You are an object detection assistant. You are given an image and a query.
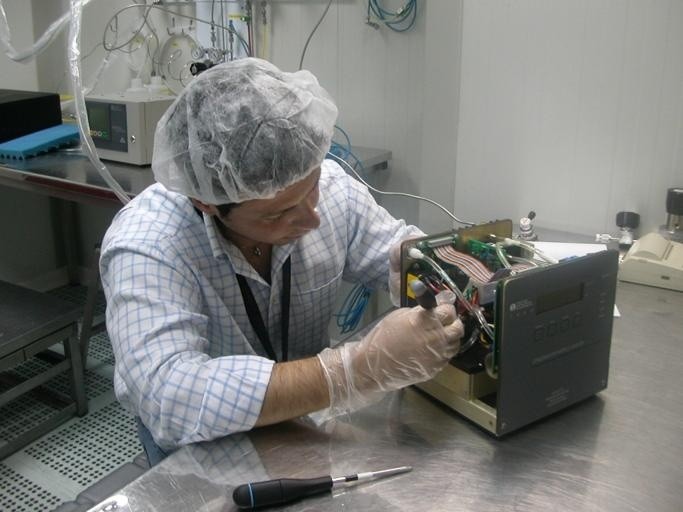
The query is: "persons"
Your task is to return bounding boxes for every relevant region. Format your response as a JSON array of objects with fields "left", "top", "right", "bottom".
[
  {"left": 148, "top": 430, "right": 314, "bottom": 485},
  {"left": 96, "top": 56, "right": 470, "bottom": 456}
]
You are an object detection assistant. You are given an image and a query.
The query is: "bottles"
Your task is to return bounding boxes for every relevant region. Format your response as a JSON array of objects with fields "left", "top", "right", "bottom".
[
  {"left": 516, "top": 217, "right": 538, "bottom": 242},
  {"left": 149, "top": 76, "right": 167, "bottom": 96},
  {"left": 127, "top": 79, "right": 148, "bottom": 99}
]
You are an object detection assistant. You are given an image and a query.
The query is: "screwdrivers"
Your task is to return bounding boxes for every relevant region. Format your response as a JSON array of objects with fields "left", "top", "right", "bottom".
[{"left": 232, "top": 463, "right": 414, "bottom": 509}]
